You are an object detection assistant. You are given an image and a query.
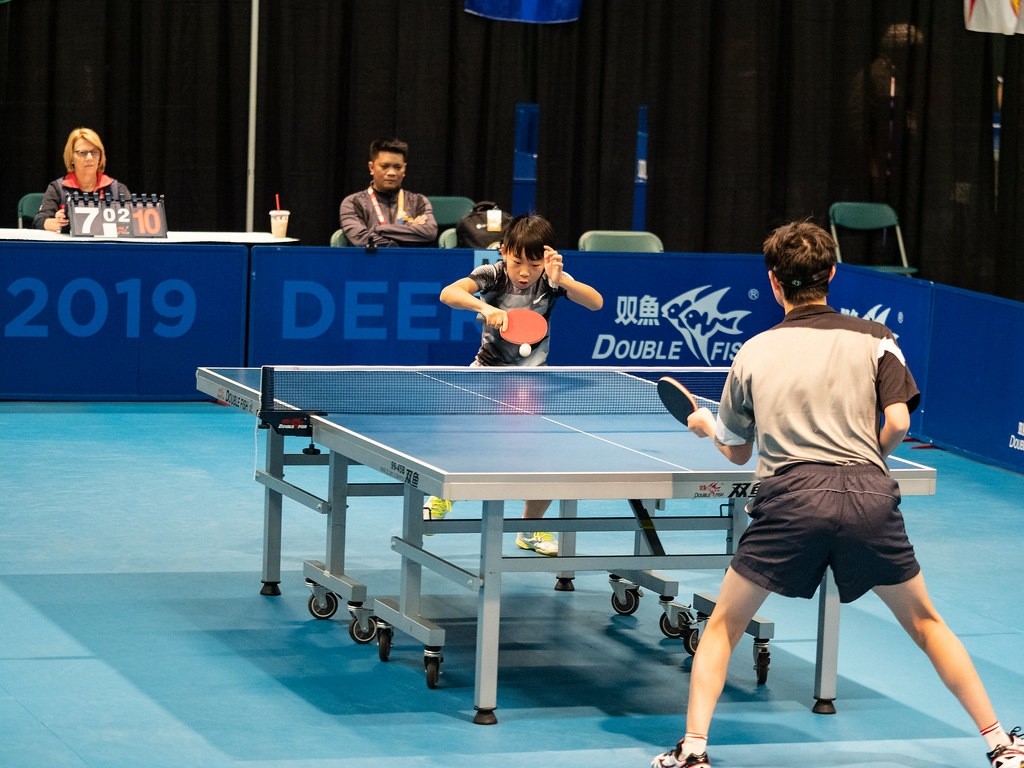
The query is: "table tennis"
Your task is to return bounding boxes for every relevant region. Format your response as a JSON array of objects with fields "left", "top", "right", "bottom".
[{"left": 519, "top": 343, "right": 532, "bottom": 358}]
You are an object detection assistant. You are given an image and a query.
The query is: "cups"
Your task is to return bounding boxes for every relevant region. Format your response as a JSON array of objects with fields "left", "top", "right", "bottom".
[
  {"left": 487, "top": 210, "right": 502, "bottom": 230},
  {"left": 269, "top": 210, "right": 290, "bottom": 238}
]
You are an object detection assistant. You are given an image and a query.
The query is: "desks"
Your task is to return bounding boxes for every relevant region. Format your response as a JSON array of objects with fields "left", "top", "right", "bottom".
[{"left": 197, "top": 365, "right": 938, "bottom": 726}]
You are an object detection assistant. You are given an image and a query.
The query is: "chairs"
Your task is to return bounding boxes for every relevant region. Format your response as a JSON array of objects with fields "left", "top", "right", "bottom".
[
  {"left": 829, "top": 201, "right": 918, "bottom": 277},
  {"left": 19, "top": 193, "right": 46, "bottom": 229},
  {"left": 329, "top": 197, "right": 475, "bottom": 248},
  {"left": 579, "top": 231, "right": 664, "bottom": 253}
]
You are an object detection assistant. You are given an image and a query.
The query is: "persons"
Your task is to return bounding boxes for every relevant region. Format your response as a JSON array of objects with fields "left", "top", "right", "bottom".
[
  {"left": 423, "top": 214, "right": 603, "bottom": 556},
  {"left": 340, "top": 138, "right": 438, "bottom": 247},
  {"left": 650, "top": 221, "right": 1024, "bottom": 768},
  {"left": 33, "top": 127, "right": 131, "bottom": 233}
]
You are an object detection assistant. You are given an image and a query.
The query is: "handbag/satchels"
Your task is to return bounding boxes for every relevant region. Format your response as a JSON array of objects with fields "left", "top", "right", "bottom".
[{"left": 456, "top": 201, "right": 514, "bottom": 250}]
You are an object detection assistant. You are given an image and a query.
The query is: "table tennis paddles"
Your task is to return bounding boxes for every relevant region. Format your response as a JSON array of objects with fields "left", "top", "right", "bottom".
[
  {"left": 475, "top": 308, "right": 549, "bottom": 345},
  {"left": 656, "top": 375, "right": 699, "bottom": 428}
]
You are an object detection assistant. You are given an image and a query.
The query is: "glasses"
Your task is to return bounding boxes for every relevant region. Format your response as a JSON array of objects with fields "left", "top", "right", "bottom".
[{"left": 73, "top": 150, "right": 100, "bottom": 158}]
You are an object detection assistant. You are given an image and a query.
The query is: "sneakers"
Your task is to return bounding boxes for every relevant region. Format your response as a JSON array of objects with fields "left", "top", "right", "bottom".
[
  {"left": 422, "top": 495, "right": 457, "bottom": 536},
  {"left": 516, "top": 531, "right": 558, "bottom": 556},
  {"left": 987, "top": 726, "right": 1024, "bottom": 768},
  {"left": 651, "top": 739, "right": 711, "bottom": 768}
]
